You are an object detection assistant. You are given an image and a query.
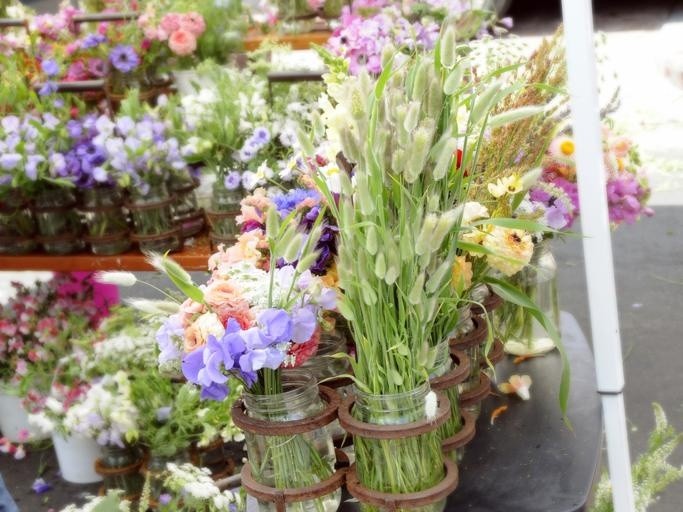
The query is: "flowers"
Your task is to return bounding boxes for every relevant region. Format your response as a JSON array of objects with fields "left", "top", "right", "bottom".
[{"left": 1, "top": 0, "right": 681, "bottom": 511}]
